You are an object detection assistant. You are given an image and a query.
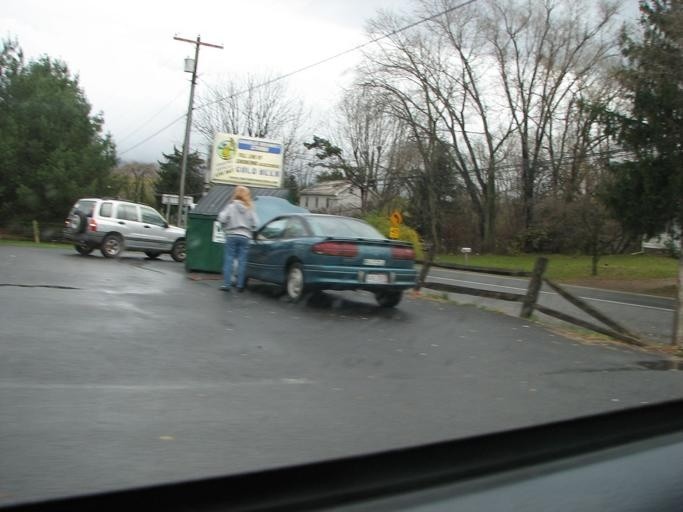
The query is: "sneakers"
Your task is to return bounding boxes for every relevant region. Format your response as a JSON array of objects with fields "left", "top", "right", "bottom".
[{"left": 219, "top": 285, "right": 244, "bottom": 293}]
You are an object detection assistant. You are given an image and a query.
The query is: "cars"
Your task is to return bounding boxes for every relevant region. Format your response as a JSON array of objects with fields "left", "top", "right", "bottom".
[{"left": 224, "top": 193, "right": 420, "bottom": 311}]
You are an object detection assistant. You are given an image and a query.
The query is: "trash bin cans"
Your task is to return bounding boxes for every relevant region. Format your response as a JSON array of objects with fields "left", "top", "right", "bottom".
[{"left": 187, "top": 185, "right": 295, "bottom": 275}]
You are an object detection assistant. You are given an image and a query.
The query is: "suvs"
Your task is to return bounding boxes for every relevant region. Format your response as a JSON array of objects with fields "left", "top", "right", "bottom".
[{"left": 63, "top": 197, "right": 191, "bottom": 262}]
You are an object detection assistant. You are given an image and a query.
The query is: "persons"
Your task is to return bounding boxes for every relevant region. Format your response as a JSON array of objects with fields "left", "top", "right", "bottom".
[{"left": 216, "top": 186, "right": 259, "bottom": 293}]
